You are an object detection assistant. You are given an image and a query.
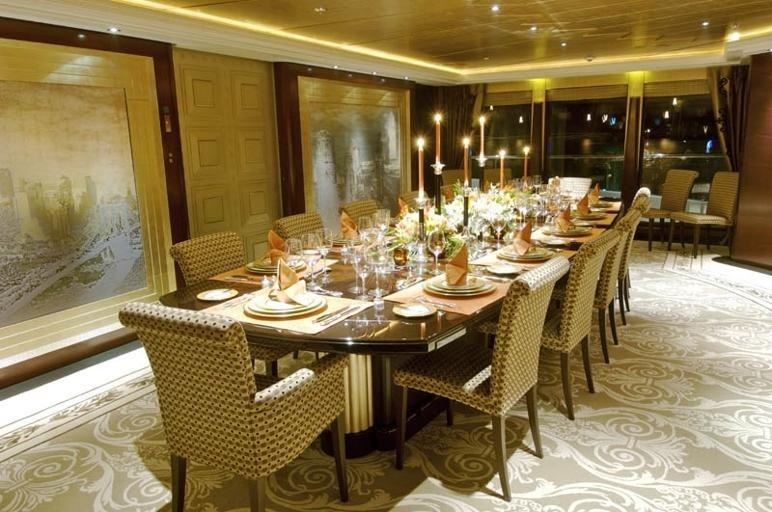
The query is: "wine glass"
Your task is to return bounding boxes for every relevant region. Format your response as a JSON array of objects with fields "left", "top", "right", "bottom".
[{"left": 300, "top": 176, "right": 565, "bottom": 304}]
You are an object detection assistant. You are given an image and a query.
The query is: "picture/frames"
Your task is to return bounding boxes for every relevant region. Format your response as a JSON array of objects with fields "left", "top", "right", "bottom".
[
  {"left": 271, "top": 59, "right": 418, "bottom": 218},
  {"left": 1, "top": 15, "right": 193, "bottom": 389}
]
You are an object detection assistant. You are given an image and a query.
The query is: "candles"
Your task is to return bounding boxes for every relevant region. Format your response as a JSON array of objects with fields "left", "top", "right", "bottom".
[{"left": 411, "top": 110, "right": 531, "bottom": 199}]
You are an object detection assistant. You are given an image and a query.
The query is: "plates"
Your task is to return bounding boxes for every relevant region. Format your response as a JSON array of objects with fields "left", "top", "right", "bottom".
[
  {"left": 423, "top": 198, "right": 611, "bottom": 299},
  {"left": 391, "top": 300, "right": 437, "bottom": 322},
  {"left": 197, "top": 232, "right": 359, "bottom": 320}
]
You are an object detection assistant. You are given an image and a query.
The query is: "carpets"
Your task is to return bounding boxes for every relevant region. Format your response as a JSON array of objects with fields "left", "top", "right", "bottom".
[{"left": 2, "top": 238, "right": 770, "bottom": 512}]
